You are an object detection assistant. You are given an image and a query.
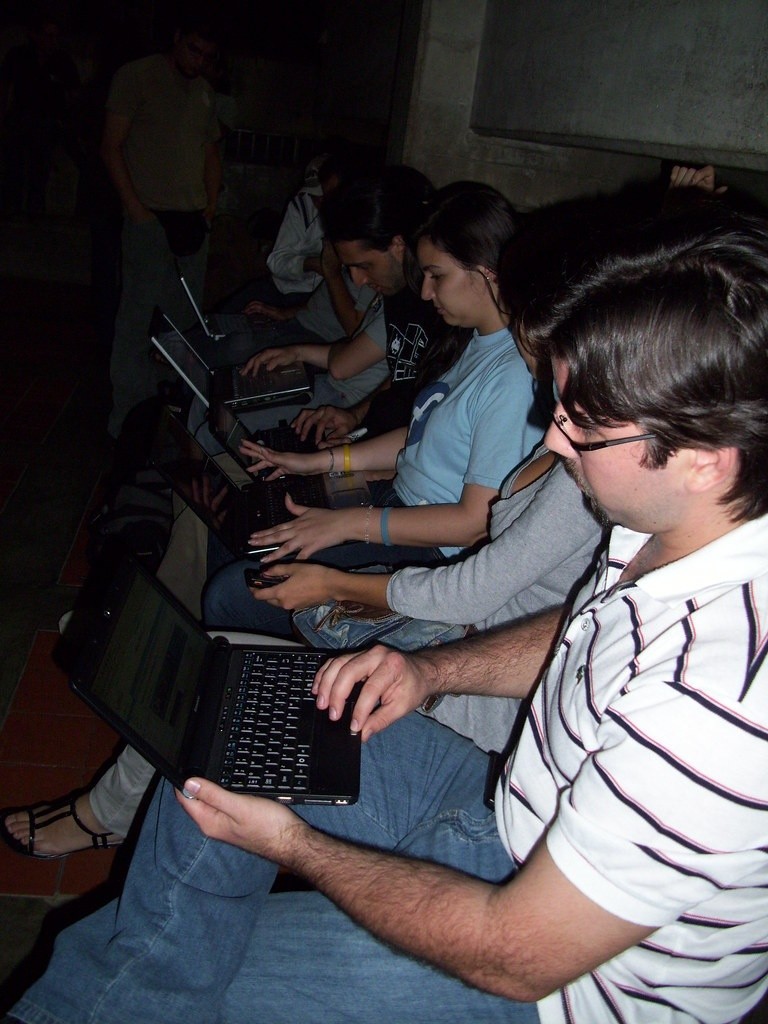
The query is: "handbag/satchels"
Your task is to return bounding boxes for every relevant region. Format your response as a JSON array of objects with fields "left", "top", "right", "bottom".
[{"left": 151, "top": 209, "right": 206, "bottom": 257}]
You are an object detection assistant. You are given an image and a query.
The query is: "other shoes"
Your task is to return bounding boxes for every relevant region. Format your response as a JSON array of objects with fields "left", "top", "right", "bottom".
[{"left": 59, "top": 609, "right": 111, "bottom": 644}]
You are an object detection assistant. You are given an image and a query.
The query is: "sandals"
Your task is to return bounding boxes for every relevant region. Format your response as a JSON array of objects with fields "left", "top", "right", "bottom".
[{"left": 0, "top": 794, "right": 144, "bottom": 861}]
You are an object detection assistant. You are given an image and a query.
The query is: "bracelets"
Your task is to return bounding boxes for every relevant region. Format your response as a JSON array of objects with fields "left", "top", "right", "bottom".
[
  {"left": 326, "top": 447, "right": 334, "bottom": 473},
  {"left": 364, "top": 505, "right": 374, "bottom": 544},
  {"left": 343, "top": 444, "right": 351, "bottom": 473},
  {"left": 381, "top": 507, "right": 393, "bottom": 547}
]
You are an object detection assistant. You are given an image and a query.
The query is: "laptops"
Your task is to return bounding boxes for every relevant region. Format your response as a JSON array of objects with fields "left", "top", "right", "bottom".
[{"left": 70, "top": 249, "right": 376, "bottom": 806}]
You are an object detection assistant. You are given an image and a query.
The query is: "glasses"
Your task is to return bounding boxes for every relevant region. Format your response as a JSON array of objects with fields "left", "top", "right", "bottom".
[{"left": 532, "top": 377, "right": 661, "bottom": 459}]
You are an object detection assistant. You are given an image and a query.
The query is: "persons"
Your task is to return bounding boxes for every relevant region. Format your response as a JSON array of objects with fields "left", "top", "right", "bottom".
[
  {"left": 0, "top": 124, "right": 730, "bottom": 866},
  {"left": 103, "top": 17, "right": 221, "bottom": 440},
  {"left": 0, "top": 194, "right": 768, "bottom": 1024}
]
